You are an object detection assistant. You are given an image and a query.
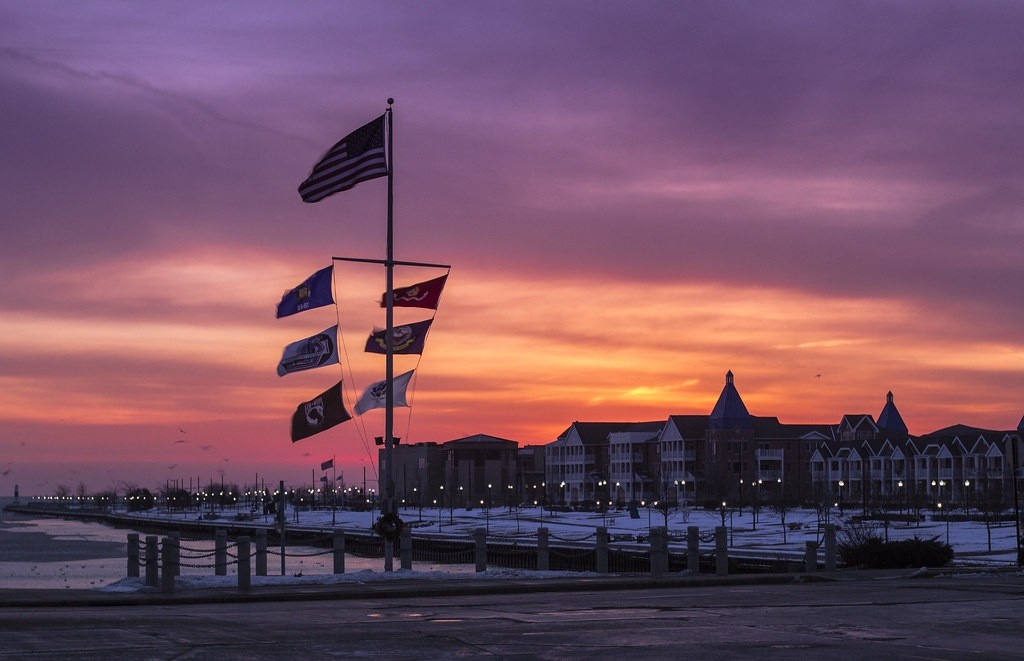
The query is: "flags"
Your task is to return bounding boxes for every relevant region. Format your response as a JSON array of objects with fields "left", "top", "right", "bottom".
[
  {"left": 364, "top": 318, "right": 433, "bottom": 357},
  {"left": 336, "top": 476, "right": 343, "bottom": 480},
  {"left": 320, "top": 476, "right": 328, "bottom": 482},
  {"left": 393, "top": 437, "right": 401, "bottom": 445},
  {"left": 321, "top": 459, "right": 334, "bottom": 471},
  {"left": 374, "top": 436, "right": 384, "bottom": 445},
  {"left": 378, "top": 274, "right": 449, "bottom": 310},
  {"left": 353, "top": 368, "right": 415, "bottom": 417},
  {"left": 275, "top": 264, "right": 335, "bottom": 319},
  {"left": 297, "top": 110, "right": 389, "bottom": 203},
  {"left": 275, "top": 324, "right": 342, "bottom": 378},
  {"left": 288, "top": 380, "right": 352, "bottom": 443}
]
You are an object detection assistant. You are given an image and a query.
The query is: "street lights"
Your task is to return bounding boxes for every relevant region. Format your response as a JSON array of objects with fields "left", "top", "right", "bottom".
[
  {"left": 839, "top": 480, "right": 844, "bottom": 517},
  {"left": 508, "top": 485, "right": 513, "bottom": 514},
  {"left": 640, "top": 499, "right": 657, "bottom": 535},
  {"left": 137, "top": 496, "right": 145, "bottom": 514},
  {"left": 598, "top": 480, "right": 607, "bottom": 526},
  {"left": 932, "top": 480, "right": 936, "bottom": 513},
  {"left": 965, "top": 480, "right": 970, "bottom": 516},
  {"left": 681, "top": 480, "right": 686, "bottom": 511},
  {"left": 739, "top": 479, "right": 743, "bottom": 516},
  {"left": 557, "top": 480, "right": 565, "bottom": 507},
  {"left": 752, "top": 482, "right": 756, "bottom": 530},
  {"left": 776, "top": 477, "right": 781, "bottom": 513},
  {"left": 758, "top": 479, "right": 762, "bottom": 509},
  {"left": 722, "top": 502, "right": 726, "bottom": 526},
  {"left": 440, "top": 485, "right": 444, "bottom": 511},
  {"left": 616, "top": 482, "right": 620, "bottom": 501},
  {"left": 674, "top": 480, "right": 678, "bottom": 511},
  {"left": 898, "top": 481, "right": 903, "bottom": 521},
  {"left": 459, "top": 486, "right": 463, "bottom": 508},
  {"left": 488, "top": 483, "right": 492, "bottom": 511},
  {"left": 939, "top": 480, "right": 945, "bottom": 516},
  {"left": 166, "top": 496, "right": 176, "bottom": 515}
]
